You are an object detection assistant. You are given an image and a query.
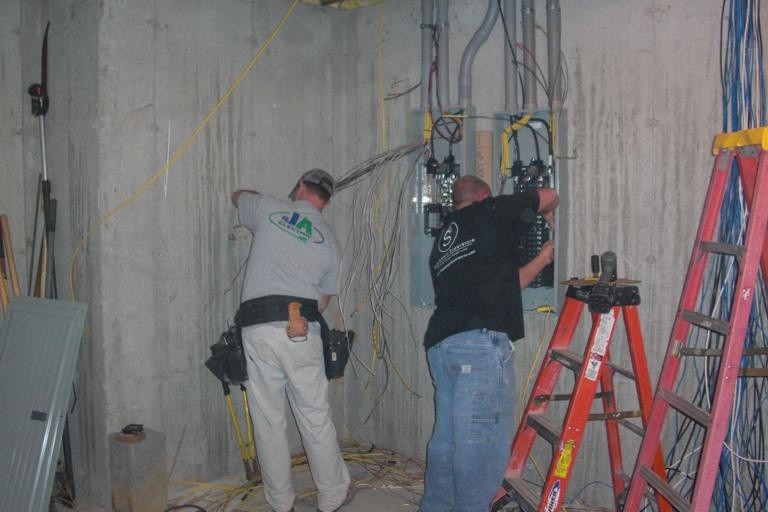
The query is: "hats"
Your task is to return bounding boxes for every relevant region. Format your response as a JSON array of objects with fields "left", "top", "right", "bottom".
[{"left": 288, "top": 169, "right": 333, "bottom": 198}]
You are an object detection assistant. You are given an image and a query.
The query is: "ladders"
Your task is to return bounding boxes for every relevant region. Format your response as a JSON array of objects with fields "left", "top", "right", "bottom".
[
  {"left": 623, "top": 127, "right": 768, "bottom": 512},
  {"left": 488, "top": 274, "right": 671, "bottom": 510}
]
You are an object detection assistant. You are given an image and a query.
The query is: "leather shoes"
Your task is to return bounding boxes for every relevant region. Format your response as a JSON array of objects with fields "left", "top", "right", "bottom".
[{"left": 317, "top": 488, "right": 353, "bottom": 512}]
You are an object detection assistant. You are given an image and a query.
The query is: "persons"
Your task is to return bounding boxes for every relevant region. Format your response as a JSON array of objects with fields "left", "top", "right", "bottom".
[
  {"left": 229, "top": 168, "right": 358, "bottom": 512},
  {"left": 417, "top": 174, "right": 561, "bottom": 511}
]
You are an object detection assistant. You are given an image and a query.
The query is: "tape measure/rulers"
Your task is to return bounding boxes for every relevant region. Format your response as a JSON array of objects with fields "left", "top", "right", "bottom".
[{"left": 123, "top": 424, "right": 143, "bottom": 433}]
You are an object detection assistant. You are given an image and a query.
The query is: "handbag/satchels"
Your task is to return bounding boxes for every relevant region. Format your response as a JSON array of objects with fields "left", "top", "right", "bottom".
[
  {"left": 323, "top": 328, "right": 354, "bottom": 382},
  {"left": 205, "top": 324, "right": 248, "bottom": 385}
]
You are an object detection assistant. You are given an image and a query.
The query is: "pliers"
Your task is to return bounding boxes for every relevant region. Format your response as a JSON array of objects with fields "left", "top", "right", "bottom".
[{"left": 222, "top": 383, "right": 261, "bottom": 485}]
